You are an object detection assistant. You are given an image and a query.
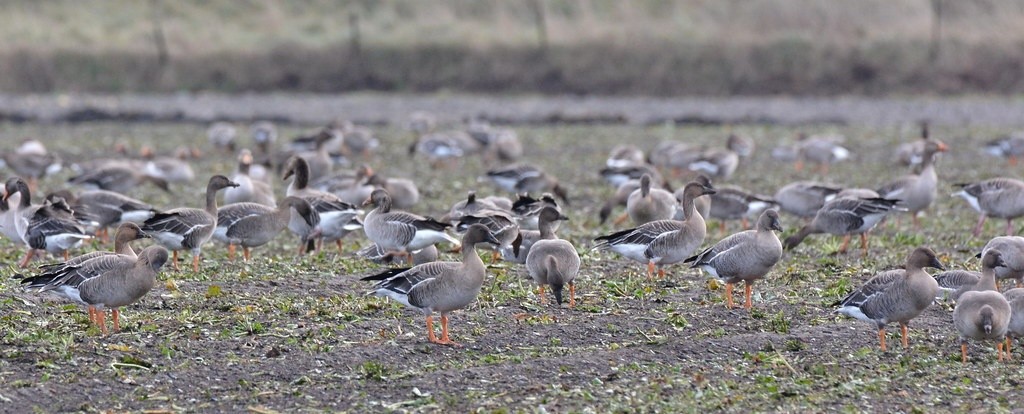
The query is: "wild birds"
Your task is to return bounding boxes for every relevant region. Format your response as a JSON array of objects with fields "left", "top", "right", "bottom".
[{"left": 0, "top": 112, "right": 1024, "bottom": 368}]
